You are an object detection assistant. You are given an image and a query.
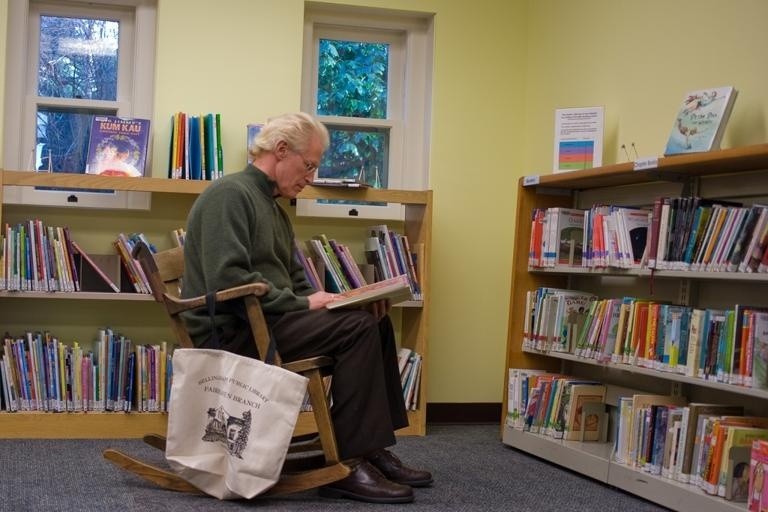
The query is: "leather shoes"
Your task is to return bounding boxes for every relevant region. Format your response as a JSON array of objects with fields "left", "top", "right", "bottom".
[
  {"left": 369, "top": 449, "right": 435, "bottom": 486},
  {"left": 319, "top": 458, "right": 415, "bottom": 503}
]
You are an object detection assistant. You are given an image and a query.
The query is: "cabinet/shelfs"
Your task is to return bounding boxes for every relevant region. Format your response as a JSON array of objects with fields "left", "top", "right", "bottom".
[
  {"left": 0, "top": 167, "right": 435, "bottom": 440},
  {"left": 499, "top": 141, "right": 768, "bottom": 512}
]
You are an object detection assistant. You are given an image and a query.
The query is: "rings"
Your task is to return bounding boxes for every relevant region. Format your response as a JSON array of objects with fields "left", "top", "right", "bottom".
[{"left": 331, "top": 294, "right": 334, "bottom": 298}]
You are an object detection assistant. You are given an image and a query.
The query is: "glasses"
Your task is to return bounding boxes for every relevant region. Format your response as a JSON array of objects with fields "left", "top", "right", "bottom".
[{"left": 300, "top": 153, "right": 319, "bottom": 178}]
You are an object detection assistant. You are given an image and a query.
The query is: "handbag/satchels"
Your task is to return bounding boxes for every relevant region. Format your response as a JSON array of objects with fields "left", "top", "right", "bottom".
[{"left": 165, "top": 348, "right": 309, "bottom": 501}]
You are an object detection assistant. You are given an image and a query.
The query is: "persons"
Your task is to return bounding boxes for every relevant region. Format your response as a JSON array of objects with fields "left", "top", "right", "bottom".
[{"left": 174, "top": 110, "right": 437, "bottom": 505}]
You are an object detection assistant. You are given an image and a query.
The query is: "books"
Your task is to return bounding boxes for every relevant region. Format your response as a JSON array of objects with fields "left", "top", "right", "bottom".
[
  {"left": 295, "top": 234, "right": 368, "bottom": 294},
  {"left": 573, "top": 297, "right": 766, "bottom": 390},
  {"left": 2, "top": 219, "right": 80, "bottom": 292},
  {"left": 523, "top": 288, "right": 599, "bottom": 352},
  {"left": 647, "top": 196, "right": 765, "bottom": 273},
  {"left": 308, "top": 176, "right": 372, "bottom": 190},
  {"left": 92, "top": 328, "right": 171, "bottom": 414},
  {"left": 114, "top": 232, "right": 157, "bottom": 295},
  {"left": 71, "top": 241, "right": 120, "bottom": 293},
  {"left": 299, "top": 375, "right": 332, "bottom": 413},
  {"left": 504, "top": 368, "right": 607, "bottom": 440},
  {"left": 582, "top": 203, "right": 652, "bottom": 268},
  {"left": 363, "top": 225, "right": 421, "bottom": 301},
  {"left": 615, "top": 394, "right": 767, "bottom": 511},
  {"left": 396, "top": 347, "right": 422, "bottom": 412},
  {"left": 528, "top": 208, "right": 585, "bottom": 268},
  {"left": 168, "top": 228, "right": 187, "bottom": 281},
  {"left": 242, "top": 120, "right": 270, "bottom": 166},
  {"left": 164, "top": 109, "right": 226, "bottom": 181},
  {"left": 80, "top": 113, "right": 151, "bottom": 180},
  {"left": 326, "top": 273, "right": 415, "bottom": 309},
  {"left": 1, "top": 331, "right": 99, "bottom": 413},
  {"left": 663, "top": 85, "right": 740, "bottom": 158},
  {"left": 358, "top": 264, "right": 375, "bottom": 284},
  {"left": 549, "top": 104, "right": 606, "bottom": 175}
]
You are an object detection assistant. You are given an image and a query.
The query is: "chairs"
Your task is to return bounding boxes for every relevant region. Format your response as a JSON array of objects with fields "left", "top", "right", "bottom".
[{"left": 102, "top": 243, "right": 351, "bottom": 500}]
